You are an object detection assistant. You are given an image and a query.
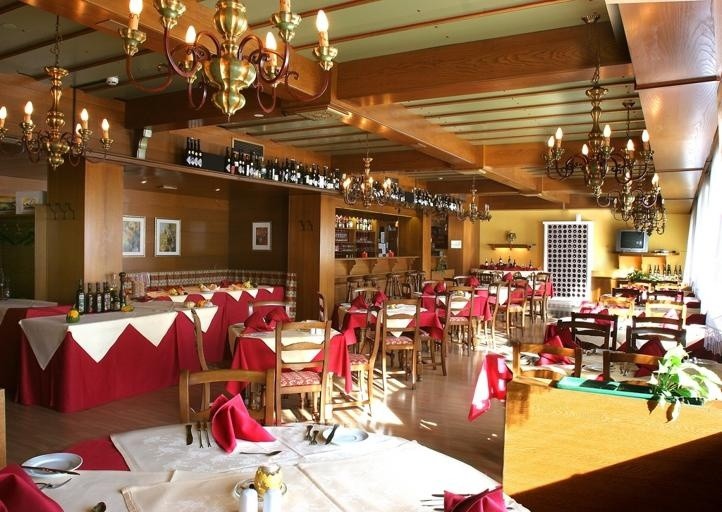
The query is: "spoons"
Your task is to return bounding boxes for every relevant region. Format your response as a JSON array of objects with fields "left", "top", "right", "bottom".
[{"left": 91, "top": 502, "right": 106, "bottom": 512}]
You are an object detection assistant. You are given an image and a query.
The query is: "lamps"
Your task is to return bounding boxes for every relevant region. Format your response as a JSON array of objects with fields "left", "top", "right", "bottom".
[
  {"left": 0, "top": 14, "right": 114, "bottom": 172},
  {"left": 456, "top": 187, "right": 492, "bottom": 223},
  {"left": 619, "top": 157, "right": 666, "bottom": 236},
  {"left": 342, "top": 133, "right": 395, "bottom": 209},
  {"left": 539, "top": 13, "right": 651, "bottom": 198},
  {"left": 596, "top": 102, "right": 660, "bottom": 217},
  {"left": 117, "top": 0, "right": 338, "bottom": 123}
]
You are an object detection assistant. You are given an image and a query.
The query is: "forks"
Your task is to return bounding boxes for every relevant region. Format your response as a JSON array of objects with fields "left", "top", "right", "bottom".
[
  {"left": 239, "top": 450, "right": 282, "bottom": 456},
  {"left": 309, "top": 430, "right": 319, "bottom": 446},
  {"left": 304, "top": 425, "right": 314, "bottom": 441}
]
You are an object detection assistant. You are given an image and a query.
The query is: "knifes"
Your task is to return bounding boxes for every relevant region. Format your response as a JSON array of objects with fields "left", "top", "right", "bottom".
[
  {"left": 21, "top": 466, "right": 80, "bottom": 475},
  {"left": 186, "top": 425, "right": 193, "bottom": 445},
  {"left": 326, "top": 424, "right": 339, "bottom": 444}
]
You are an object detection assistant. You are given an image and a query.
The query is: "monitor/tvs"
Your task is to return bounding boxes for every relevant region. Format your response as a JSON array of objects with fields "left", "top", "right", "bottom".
[{"left": 616, "top": 229, "right": 648, "bottom": 253}]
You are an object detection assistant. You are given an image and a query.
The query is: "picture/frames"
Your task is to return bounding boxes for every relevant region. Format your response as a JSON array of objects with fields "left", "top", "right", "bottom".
[
  {"left": 153, "top": 216, "right": 182, "bottom": 256},
  {"left": 251, "top": 221, "right": 273, "bottom": 252},
  {"left": 121, "top": 215, "right": 147, "bottom": 258}
]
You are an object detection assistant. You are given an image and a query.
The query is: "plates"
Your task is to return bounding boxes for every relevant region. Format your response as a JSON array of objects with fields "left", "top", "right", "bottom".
[
  {"left": 232, "top": 480, "right": 288, "bottom": 507},
  {"left": 22, "top": 453, "right": 84, "bottom": 478},
  {"left": 322, "top": 428, "right": 369, "bottom": 445}
]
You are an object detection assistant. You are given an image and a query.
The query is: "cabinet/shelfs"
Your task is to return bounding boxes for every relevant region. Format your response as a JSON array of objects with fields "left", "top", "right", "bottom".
[{"left": 334, "top": 218, "right": 378, "bottom": 258}]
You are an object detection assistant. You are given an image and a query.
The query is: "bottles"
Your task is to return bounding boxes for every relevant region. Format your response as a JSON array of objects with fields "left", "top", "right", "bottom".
[
  {"left": 224, "top": 146, "right": 464, "bottom": 260},
  {"left": 185, "top": 136, "right": 203, "bottom": 168},
  {"left": 479, "top": 255, "right": 533, "bottom": 286},
  {"left": 75, "top": 272, "right": 121, "bottom": 315},
  {"left": 649, "top": 265, "right": 682, "bottom": 281},
  {"left": 0, "top": 263, "right": 12, "bottom": 298}
]
